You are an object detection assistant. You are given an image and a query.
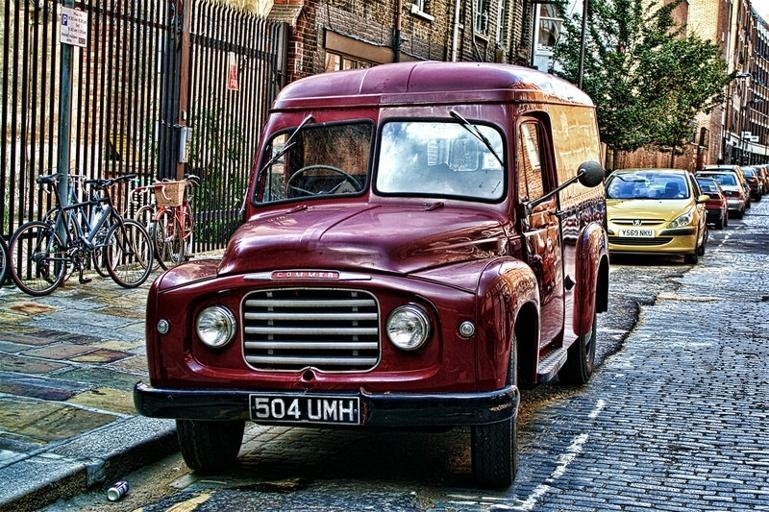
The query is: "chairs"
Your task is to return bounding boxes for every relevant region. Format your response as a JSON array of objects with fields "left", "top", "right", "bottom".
[{"left": 664, "top": 178, "right": 682, "bottom": 200}]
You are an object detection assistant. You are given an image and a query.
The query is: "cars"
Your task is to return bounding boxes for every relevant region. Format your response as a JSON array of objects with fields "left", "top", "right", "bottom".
[
  {"left": 696, "top": 177, "right": 733, "bottom": 230},
  {"left": 604, "top": 167, "right": 710, "bottom": 265},
  {"left": 694, "top": 171, "right": 749, "bottom": 219},
  {"left": 740, "top": 163, "right": 769, "bottom": 202},
  {"left": 695, "top": 163, "right": 756, "bottom": 209}
]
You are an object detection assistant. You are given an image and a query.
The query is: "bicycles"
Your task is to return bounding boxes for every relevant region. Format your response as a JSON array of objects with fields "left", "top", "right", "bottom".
[
  {"left": 151, "top": 173, "right": 200, "bottom": 272},
  {"left": 9, "top": 174, "right": 153, "bottom": 296},
  {"left": 0, "top": 235, "right": 9, "bottom": 290},
  {"left": 128, "top": 185, "right": 189, "bottom": 274},
  {"left": 36, "top": 174, "right": 124, "bottom": 287}
]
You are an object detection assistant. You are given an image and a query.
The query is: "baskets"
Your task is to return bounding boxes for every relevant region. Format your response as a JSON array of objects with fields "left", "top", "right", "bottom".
[{"left": 152, "top": 180, "right": 186, "bottom": 208}]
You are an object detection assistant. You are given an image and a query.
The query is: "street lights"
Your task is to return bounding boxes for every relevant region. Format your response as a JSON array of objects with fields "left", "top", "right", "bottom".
[
  {"left": 721, "top": 74, "right": 751, "bottom": 166},
  {"left": 740, "top": 99, "right": 764, "bottom": 165}
]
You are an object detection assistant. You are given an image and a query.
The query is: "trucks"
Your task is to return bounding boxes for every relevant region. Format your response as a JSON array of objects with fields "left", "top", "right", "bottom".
[{"left": 132, "top": 59, "right": 612, "bottom": 491}]
